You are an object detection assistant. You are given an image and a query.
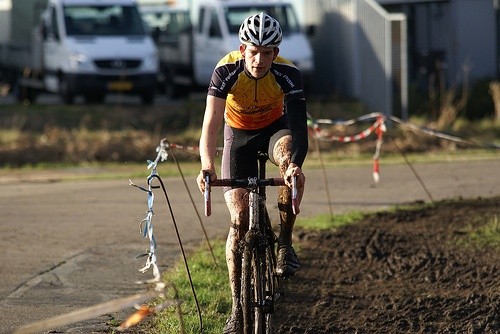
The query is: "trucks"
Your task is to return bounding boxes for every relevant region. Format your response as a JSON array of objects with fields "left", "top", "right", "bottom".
[{"left": 0, "top": 0, "right": 164, "bottom": 107}]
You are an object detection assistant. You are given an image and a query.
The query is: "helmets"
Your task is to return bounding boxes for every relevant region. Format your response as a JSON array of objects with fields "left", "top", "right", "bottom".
[{"left": 238, "top": 12, "right": 283, "bottom": 48}]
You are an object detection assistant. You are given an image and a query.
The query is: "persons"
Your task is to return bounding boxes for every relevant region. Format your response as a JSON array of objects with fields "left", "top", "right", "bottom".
[{"left": 196, "top": 12, "right": 308, "bottom": 334}]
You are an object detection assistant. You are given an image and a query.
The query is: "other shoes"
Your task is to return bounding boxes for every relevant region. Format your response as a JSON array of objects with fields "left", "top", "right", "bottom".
[
  {"left": 223, "top": 311, "right": 243, "bottom": 334},
  {"left": 275, "top": 246, "right": 300, "bottom": 276}
]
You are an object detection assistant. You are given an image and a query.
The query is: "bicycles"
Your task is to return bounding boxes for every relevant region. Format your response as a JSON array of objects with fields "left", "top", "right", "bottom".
[{"left": 204, "top": 174, "right": 300, "bottom": 334}]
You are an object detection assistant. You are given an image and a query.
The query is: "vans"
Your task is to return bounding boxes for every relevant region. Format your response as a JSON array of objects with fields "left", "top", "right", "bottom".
[{"left": 140, "top": 1, "right": 317, "bottom": 97}]
